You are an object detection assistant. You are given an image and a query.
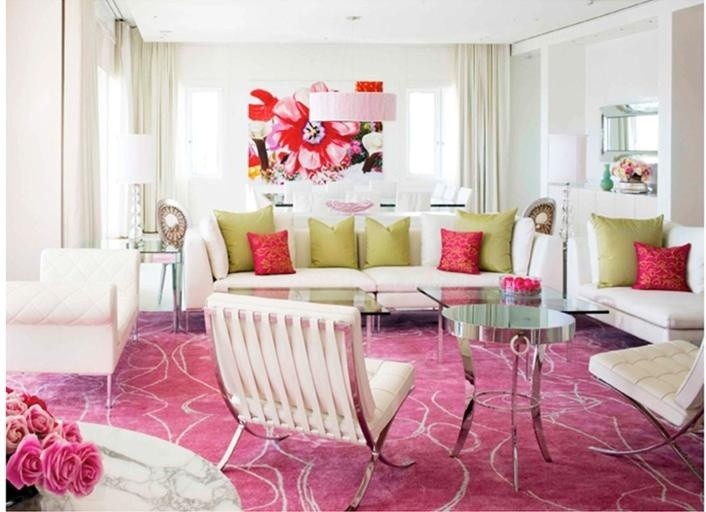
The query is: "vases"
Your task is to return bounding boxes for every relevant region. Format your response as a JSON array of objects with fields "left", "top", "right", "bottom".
[{"left": 618, "top": 181, "right": 649, "bottom": 195}]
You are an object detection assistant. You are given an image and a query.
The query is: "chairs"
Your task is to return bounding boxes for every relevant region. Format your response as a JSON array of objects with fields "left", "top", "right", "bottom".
[
  {"left": 431, "top": 182, "right": 475, "bottom": 202},
  {"left": 206, "top": 295, "right": 419, "bottom": 509},
  {"left": 590, "top": 319, "right": 702, "bottom": 486}
]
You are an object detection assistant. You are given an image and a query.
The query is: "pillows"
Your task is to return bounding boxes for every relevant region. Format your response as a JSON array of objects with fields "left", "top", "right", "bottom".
[
  {"left": 363, "top": 214, "right": 412, "bottom": 268},
  {"left": 457, "top": 209, "right": 520, "bottom": 274},
  {"left": 305, "top": 214, "right": 360, "bottom": 267},
  {"left": 510, "top": 216, "right": 535, "bottom": 277},
  {"left": 199, "top": 207, "right": 229, "bottom": 281},
  {"left": 437, "top": 227, "right": 481, "bottom": 276},
  {"left": 632, "top": 240, "right": 693, "bottom": 291},
  {"left": 246, "top": 231, "right": 296, "bottom": 274},
  {"left": 589, "top": 212, "right": 665, "bottom": 289},
  {"left": 213, "top": 203, "right": 276, "bottom": 274}
]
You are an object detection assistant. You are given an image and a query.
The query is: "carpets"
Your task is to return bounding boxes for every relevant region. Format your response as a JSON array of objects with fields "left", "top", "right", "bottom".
[{"left": 5, "top": 309, "right": 703, "bottom": 512}]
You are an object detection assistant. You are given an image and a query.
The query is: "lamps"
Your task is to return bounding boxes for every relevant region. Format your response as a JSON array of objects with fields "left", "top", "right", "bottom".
[
  {"left": 117, "top": 133, "right": 152, "bottom": 240},
  {"left": 308, "top": 90, "right": 399, "bottom": 200},
  {"left": 546, "top": 134, "right": 585, "bottom": 237}
]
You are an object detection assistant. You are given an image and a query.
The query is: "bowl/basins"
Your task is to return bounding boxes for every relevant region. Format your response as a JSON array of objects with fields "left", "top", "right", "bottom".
[{"left": 497, "top": 277, "right": 543, "bottom": 297}]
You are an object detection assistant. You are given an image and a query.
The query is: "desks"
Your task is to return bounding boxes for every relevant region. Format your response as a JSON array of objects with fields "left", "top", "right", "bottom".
[
  {"left": 227, "top": 287, "right": 390, "bottom": 357},
  {"left": 137, "top": 245, "right": 181, "bottom": 333},
  {"left": 416, "top": 283, "right": 611, "bottom": 364},
  {"left": 40, "top": 418, "right": 245, "bottom": 512},
  {"left": 441, "top": 303, "right": 574, "bottom": 490}
]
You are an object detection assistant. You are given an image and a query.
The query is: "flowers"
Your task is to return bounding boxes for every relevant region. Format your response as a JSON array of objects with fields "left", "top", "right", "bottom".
[
  {"left": 4, "top": 379, "right": 105, "bottom": 512},
  {"left": 611, "top": 155, "right": 651, "bottom": 181}
]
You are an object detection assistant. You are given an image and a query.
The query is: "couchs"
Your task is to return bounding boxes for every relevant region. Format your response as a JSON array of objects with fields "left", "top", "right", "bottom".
[
  {"left": 571, "top": 221, "right": 703, "bottom": 342},
  {"left": 182, "top": 202, "right": 561, "bottom": 333},
  {"left": 5, "top": 242, "right": 143, "bottom": 408}
]
